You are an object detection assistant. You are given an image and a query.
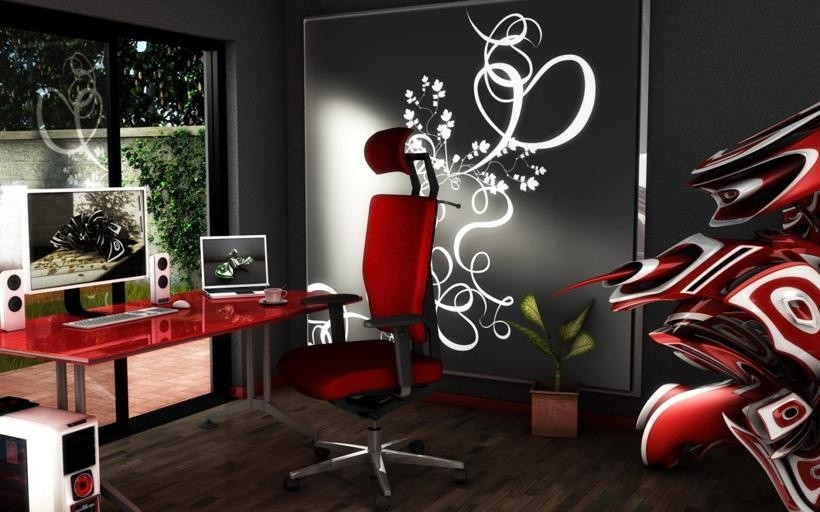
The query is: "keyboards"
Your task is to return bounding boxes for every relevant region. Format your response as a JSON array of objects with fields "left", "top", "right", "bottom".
[{"left": 61, "top": 307, "right": 179, "bottom": 329}]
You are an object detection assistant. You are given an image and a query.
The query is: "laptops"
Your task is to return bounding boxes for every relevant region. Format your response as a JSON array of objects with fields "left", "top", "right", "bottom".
[{"left": 199, "top": 234, "right": 270, "bottom": 300}]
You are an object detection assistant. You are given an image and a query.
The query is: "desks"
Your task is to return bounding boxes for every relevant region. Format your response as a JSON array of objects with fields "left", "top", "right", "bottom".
[{"left": 0, "top": 286, "right": 362, "bottom": 512}]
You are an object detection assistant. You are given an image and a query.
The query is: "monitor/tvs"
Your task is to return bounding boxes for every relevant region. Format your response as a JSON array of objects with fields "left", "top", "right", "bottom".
[{"left": 21, "top": 186, "right": 148, "bottom": 317}]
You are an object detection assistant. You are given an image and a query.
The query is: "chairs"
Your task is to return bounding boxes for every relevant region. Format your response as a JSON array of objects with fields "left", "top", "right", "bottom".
[{"left": 273, "top": 125, "right": 470, "bottom": 510}]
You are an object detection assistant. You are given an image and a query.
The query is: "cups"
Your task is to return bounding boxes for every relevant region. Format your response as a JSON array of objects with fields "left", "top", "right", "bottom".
[{"left": 264, "top": 288, "right": 287, "bottom": 303}]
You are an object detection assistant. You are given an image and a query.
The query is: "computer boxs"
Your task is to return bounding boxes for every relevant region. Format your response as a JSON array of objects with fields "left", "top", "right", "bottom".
[{"left": 0, "top": 406, "right": 101, "bottom": 512}]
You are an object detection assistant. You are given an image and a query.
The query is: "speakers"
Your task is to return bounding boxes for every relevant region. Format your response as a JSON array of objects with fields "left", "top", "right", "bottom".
[
  {"left": 149, "top": 253, "right": 170, "bottom": 304},
  {"left": 0, "top": 269, "right": 26, "bottom": 332}
]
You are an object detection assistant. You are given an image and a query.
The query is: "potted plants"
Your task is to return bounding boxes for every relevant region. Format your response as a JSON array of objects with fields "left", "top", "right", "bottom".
[{"left": 506, "top": 289, "right": 597, "bottom": 440}]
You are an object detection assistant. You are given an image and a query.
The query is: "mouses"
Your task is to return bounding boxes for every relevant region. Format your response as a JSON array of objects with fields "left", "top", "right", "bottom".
[{"left": 172, "top": 299, "right": 191, "bottom": 308}]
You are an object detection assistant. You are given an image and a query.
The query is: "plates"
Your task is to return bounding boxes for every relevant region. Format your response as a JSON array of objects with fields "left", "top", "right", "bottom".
[{"left": 258, "top": 298, "right": 288, "bottom": 305}]
